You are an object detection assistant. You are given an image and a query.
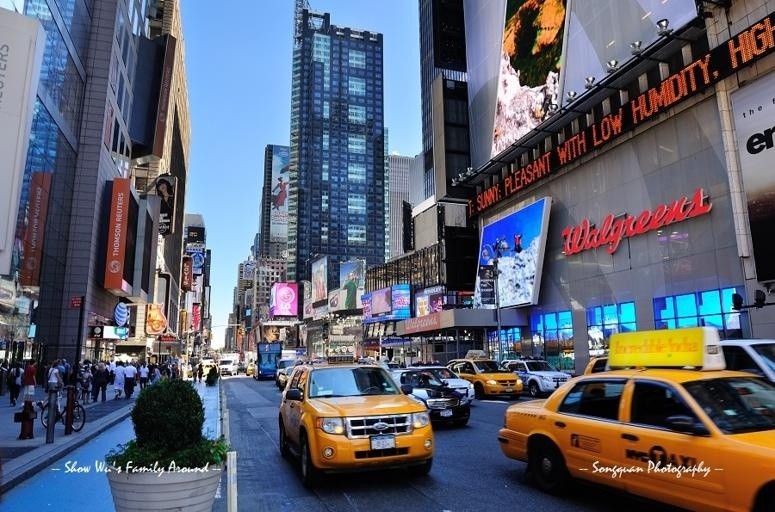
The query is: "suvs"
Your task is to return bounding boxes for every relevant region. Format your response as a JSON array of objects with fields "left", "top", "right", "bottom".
[
  {"left": 584, "top": 353, "right": 609, "bottom": 376},
  {"left": 278, "top": 354, "right": 435, "bottom": 487},
  {"left": 498, "top": 355, "right": 573, "bottom": 398}
]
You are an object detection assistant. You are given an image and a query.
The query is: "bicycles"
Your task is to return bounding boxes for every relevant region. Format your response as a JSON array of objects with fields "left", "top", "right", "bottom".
[{"left": 41, "top": 386, "right": 87, "bottom": 432}]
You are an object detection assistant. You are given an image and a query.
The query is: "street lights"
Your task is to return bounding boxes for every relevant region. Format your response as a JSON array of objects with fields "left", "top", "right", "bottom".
[{"left": 482, "top": 235, "right": 509, "bottom": 364}]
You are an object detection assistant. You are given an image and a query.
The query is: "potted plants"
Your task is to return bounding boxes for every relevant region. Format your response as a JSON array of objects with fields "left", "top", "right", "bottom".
[{"left": 104, "top": 377, "right": 231, "bottom": 512}]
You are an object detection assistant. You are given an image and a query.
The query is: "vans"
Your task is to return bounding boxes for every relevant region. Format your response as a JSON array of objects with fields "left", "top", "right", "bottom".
[{"left": 605, "top": 338, "right": 775, "bottom": 383}]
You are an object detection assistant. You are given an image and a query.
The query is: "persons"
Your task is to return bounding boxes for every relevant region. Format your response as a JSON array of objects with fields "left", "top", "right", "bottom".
[
  {"left": 342, "top": 271, "right": 361, "bottom": 309},
  {"left": 313, "top": 264, "right": 326, "bottom": 300},
  {"left": 271, "top": 174, "right": 289, "bottom": 211},
  {"left": 155, "top": 179, "right": 174, "bottom": 233},
  {"left": 0, "top": 352, "right": 206, "bottom": 413}
]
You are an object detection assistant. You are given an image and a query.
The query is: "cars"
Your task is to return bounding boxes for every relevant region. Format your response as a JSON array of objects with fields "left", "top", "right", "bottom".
[
  {"left": 147, "top": 340, "right": 420, "bottom": 393},
  {"left": 443, "top": 350, "right": 523, "bottom": 400},
  {"left": 405, "top": 361, "right": 475, "bottom": 405},
  {"left": 498, "top": 326, "right": 775, "bottom": 512},
  {"left": 385, "top": 369, "right": 470, "bottom": 428}
]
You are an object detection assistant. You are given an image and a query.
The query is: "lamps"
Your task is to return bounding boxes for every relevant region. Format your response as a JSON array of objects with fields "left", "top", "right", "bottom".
[
  {"left": 732, "top": 290, "right": 775, "bottom": 310},
  {"left": 452, "top": 167, "right": 474, "bottom": 185},
  {"left": 548, "top": 19, "right": 673, "bottom": 117}
]
